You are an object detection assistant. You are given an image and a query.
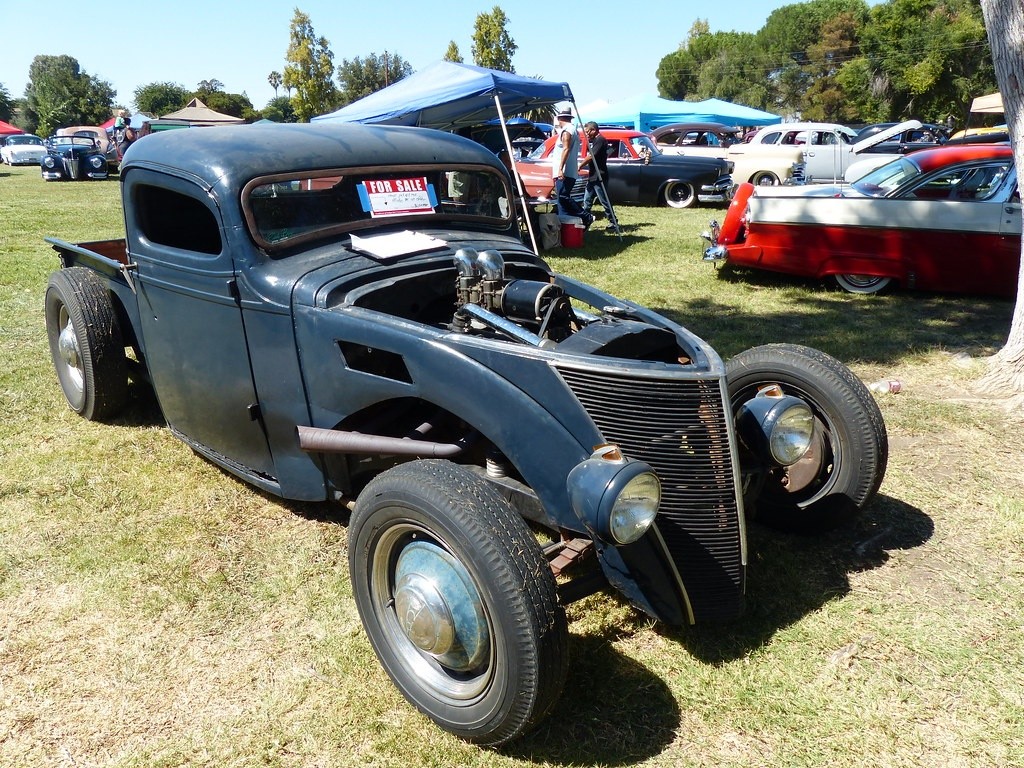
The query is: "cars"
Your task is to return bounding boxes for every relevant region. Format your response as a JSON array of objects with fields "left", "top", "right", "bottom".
[
  {"left": 448, "top": 116, "right": 1013, "bottom": 210},
  {"left": 39, "top": 134, "right": 109, "bottom": 181},
  {"left": 53, "top": 124, "right": 119, "bottom": 166},
  {"left": 701, "top": 143, "right": 1022, "bottom": 304},
  {"left": 0, "top": 133, "right": 47, "bottom": 167}
]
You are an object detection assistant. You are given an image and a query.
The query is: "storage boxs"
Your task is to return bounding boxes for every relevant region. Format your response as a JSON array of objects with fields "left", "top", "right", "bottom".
[{"left": 558, "top": 214, "right": 585, "bottom": 247}]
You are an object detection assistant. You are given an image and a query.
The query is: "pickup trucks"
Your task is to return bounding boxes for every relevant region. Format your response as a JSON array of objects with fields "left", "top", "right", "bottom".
[{"left": 39, "top": 120, "right": 891, "bottom": 749}]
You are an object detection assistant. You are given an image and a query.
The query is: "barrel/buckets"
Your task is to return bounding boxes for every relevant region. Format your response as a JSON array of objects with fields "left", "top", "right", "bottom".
[{"left": 561, "top": 217, "right": 586, "bottom": 249}]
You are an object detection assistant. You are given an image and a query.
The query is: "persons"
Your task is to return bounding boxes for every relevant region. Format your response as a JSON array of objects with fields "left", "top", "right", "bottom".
[
  {"left": 581, "top": 121, "right": 620, "bottom": 230},
  {"left": 114, "top": 118, "right": 137, "bottom": 164},
  {"left": 137, "top": 121, "right": 152, "bottom": 138},
  {"left": 115, "top": 110, "right": 133, "bottom": 131},
  {"left": 552, "top": 107, "right": 595, "bottom": 231}
]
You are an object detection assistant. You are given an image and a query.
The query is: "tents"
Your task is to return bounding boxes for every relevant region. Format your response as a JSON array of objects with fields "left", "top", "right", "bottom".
[
  {"left": 147, "top": 97, "right": 246, "bottom": 132},
  {"left": 0, "top": 120, "right": 25, "bottom": 146},
  {"left": 573, "top": 95, "right": 781, "bottom": 148},
  {"left": 310, "top": 61, "right": 625, "bottom": 257},
  {"left": 99, "top": 113, "right": 152, "bottom": 134}
]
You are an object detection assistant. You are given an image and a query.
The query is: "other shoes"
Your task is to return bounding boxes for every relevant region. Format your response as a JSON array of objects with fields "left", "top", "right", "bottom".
[
  {"left": 584, "top": 216, "right": 596, "bottom": 231},
  {"left": 605, "top": 222, "right": 620, "bottom": 231}
]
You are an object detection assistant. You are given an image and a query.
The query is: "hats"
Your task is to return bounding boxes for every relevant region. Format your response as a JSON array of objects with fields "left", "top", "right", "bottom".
[{"left": 556, "top": 106, "right": 575, "bottom": 119}]
